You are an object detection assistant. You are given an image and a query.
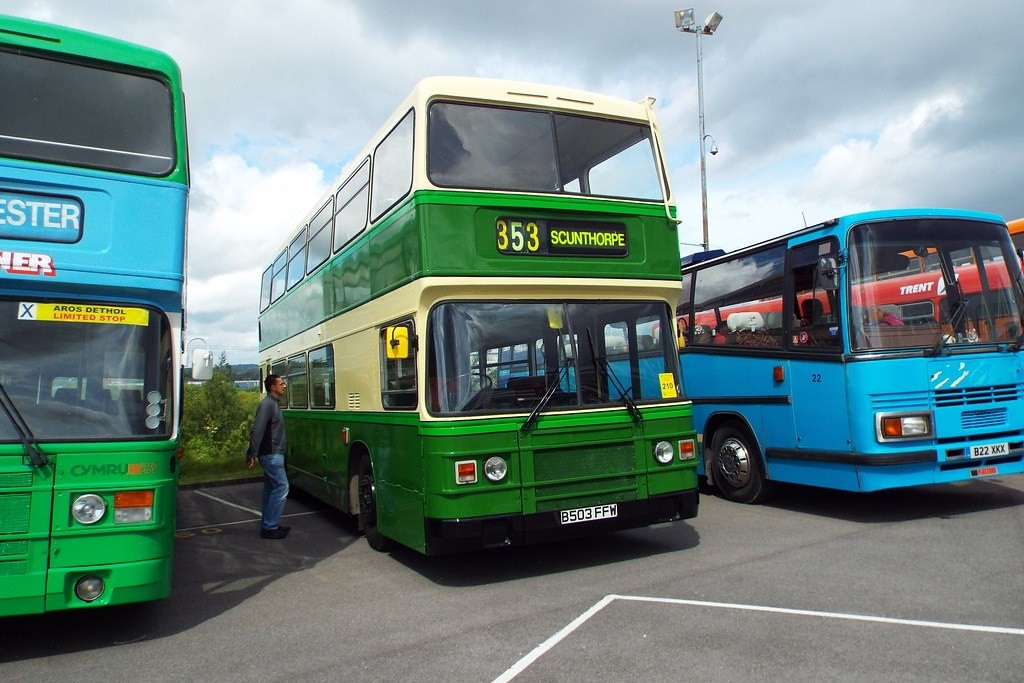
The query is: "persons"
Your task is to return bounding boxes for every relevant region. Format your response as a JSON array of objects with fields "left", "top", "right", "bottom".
[{"left": 246, "top": 375, "right": 291, "bottom": 539}]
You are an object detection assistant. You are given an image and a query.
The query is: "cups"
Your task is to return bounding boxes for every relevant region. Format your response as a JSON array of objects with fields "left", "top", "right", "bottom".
[{"left": 943, "top": 335, "right": 955, "bottom": 344}]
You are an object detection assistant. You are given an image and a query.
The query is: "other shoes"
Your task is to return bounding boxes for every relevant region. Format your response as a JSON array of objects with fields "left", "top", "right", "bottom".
[{"left": 262, "top": 526, "right": 291, "bottom": 540}]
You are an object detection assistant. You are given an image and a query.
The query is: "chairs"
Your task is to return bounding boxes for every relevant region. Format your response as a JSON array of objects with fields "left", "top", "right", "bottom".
[
  {"left": 634, "top": 310, "right": 801, "bottom": 346},
  {"left": 508, "top": 374, "right": 548, "bottom": 396},
  {"left": 801, "top": 299, "right": 827, "bottom": 324},
  {"left": 399, "top": 372, "right": 417, "bottom": 406},
  {"left": 291, "top": 382, "right": 334, "bottom": 407},
  {"left": 55, "top": 388, "right": 143, "bottom": 402}
]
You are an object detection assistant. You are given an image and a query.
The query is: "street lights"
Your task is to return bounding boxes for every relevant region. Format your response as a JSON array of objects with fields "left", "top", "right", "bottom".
[{"left": 674, "top": 8, "right": 724, "bottom": 252}]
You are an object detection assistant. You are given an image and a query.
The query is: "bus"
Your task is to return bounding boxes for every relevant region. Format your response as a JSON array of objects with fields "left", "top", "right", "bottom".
[
  {"left": 497, "top": 208, "right": 1024, "bottom": 505},
  {"left": 871, "top": 218, "right": 1024, "bottom": 267},
  {"left": 257, "top": 76, "right": 701, "bottom": 558},
  {"left": 0, "top": 15, "right": 217, "bottom": 617},
  {"left": 652, "top": 258, "right": 1024, "bottom": 344}
]
